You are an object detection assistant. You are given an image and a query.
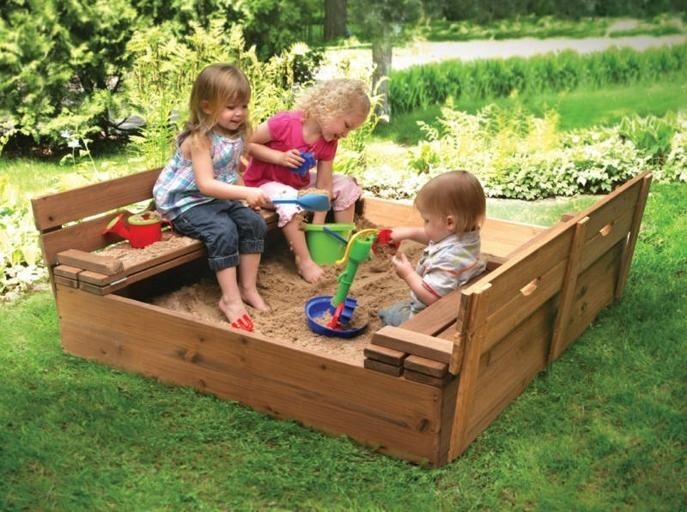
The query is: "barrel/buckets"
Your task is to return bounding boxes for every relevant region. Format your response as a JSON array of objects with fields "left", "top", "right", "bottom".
[{"left": 307, "top": 222, "right": 355, "bottom": 263}]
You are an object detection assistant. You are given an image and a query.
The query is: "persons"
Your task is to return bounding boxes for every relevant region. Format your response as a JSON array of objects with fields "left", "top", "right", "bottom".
[
  {"left": 242, "top": 78, "right": 372, "bottom": 284},
  {"left": 153, "top": 63, "right": 273, "bottom": 322},
  {"left": 380, "top": 170, "right": 485, "bottom": 326}
]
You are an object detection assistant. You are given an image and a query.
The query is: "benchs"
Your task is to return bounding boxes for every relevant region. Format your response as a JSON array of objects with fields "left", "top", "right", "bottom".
[
  {"left": 31, "top": 166, "right": 277, "bottom": 354},
  {"left": 363, "top": 168, "right": 654, "bottom": 470}
]
